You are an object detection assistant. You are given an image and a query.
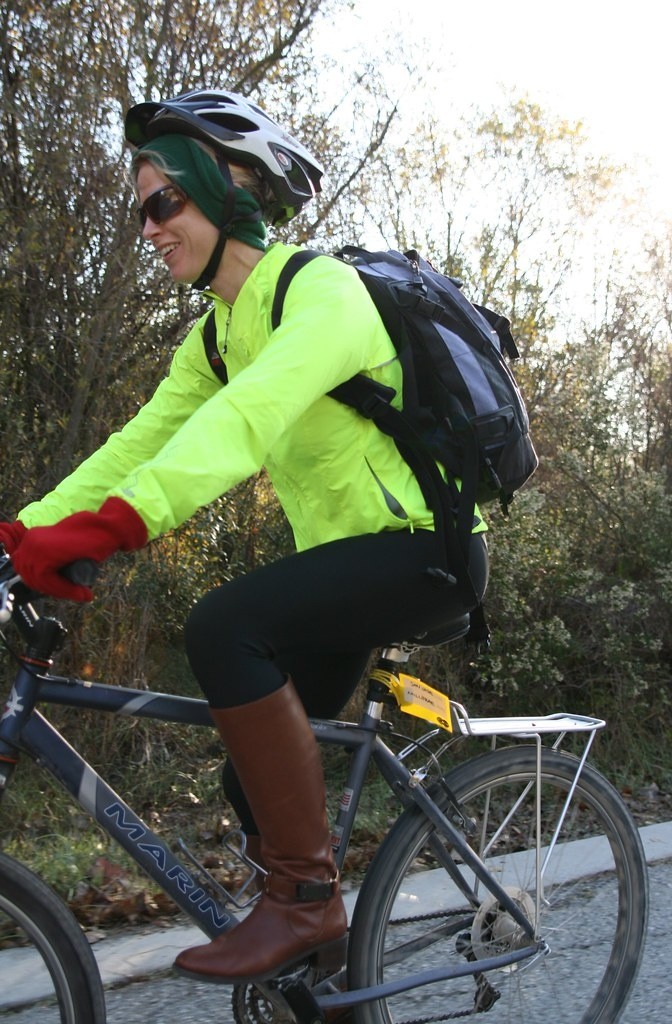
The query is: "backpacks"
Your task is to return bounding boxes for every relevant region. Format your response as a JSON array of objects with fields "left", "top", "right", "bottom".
[{"left": 202, "top": 245, "right": 539, "bottom": 515}]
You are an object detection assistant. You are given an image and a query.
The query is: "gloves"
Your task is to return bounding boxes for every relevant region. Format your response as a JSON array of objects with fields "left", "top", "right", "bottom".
[
  {"left": 0, "top": 519, "right": 30, "bottom": 556},
  {"left": 12, "top": 496, "right": 148, "bottom": 603}
]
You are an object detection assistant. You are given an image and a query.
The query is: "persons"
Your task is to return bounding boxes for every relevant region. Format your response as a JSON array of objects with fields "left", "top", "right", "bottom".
[{"left": 0, "top": 90, "right": 488, "bottom": 983}]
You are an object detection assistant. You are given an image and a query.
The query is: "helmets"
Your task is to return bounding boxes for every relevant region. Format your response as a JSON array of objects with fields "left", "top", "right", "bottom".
[{"left": 125, "top": 90, "right": 324, "bottom": 229}]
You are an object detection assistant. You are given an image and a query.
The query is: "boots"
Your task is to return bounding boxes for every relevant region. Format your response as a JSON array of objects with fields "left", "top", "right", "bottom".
[{"left": 172, "top": 673, "right": 350, "bottom": 1024}]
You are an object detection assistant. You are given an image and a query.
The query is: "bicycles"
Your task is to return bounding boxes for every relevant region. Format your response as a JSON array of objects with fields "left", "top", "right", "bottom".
[{"left": 0, "top": 546, "right": 651, "bottom": 1024}]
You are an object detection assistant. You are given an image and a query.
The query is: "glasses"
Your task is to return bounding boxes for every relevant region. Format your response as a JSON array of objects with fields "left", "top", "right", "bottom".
[{"left": 136, "top": 185, "right": 187, "bottom": 230}]
